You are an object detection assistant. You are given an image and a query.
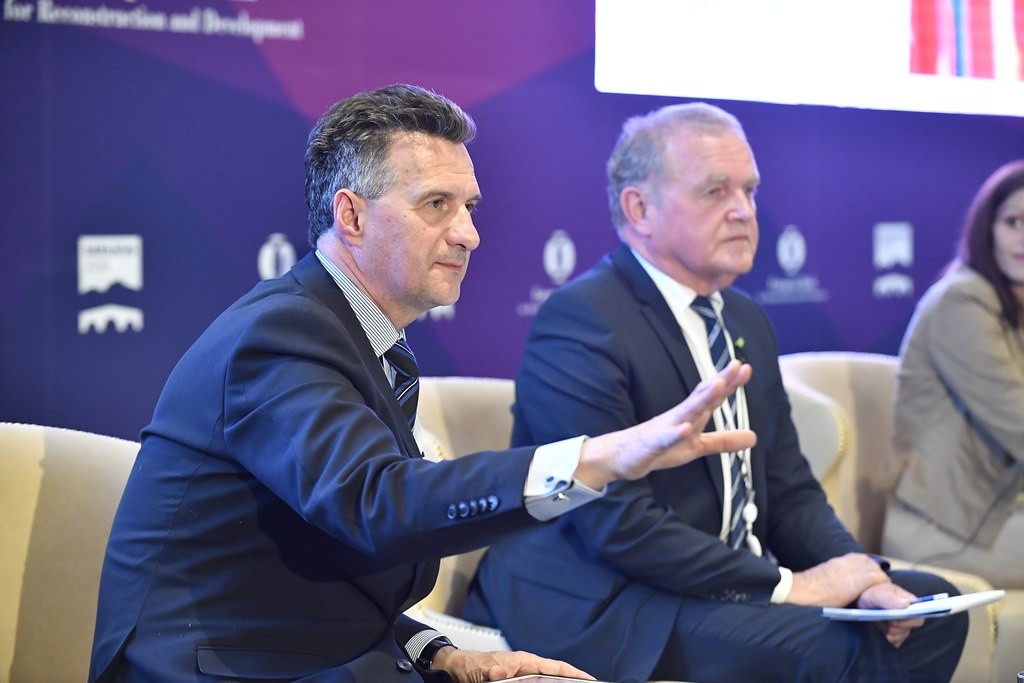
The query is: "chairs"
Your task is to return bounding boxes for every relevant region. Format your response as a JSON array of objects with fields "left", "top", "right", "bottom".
[
  {"left": 0, "top": 421, "right": 147, "bottom": 683},
  {"left": 779, "top": 350, "right": 998, "bottom": 683},
  {"left": 410, "top": 377, "right": 518, "bottom": 658}
]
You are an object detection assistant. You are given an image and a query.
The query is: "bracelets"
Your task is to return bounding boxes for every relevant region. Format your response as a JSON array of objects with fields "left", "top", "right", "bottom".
[{"left": 416, "top": 639, "right": 458, "bottom": 670}]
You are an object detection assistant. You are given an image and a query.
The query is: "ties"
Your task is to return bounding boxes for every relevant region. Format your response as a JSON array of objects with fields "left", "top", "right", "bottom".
[
  {"left": 385, "top": 339, "right": 419, "bottom": 433},
  {"left": 691, "top": 296, "right": 749, "bottom": 600}
]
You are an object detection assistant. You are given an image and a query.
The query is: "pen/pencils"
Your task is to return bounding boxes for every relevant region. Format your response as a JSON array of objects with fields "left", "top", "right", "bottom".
[{"left": 909, "top": 593, "right": 949, "bottom": 605}]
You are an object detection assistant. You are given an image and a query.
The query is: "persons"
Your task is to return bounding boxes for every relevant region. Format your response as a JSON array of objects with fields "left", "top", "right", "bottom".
[
  {"left": 464, "top": 102, "right": 969, "bottom": 683},
  {"left": 881, "top": 161, "right": 1024, "bottom": 591},
  {"left": 86, "top": 83, "right": 757, "bottom": 682}
]
